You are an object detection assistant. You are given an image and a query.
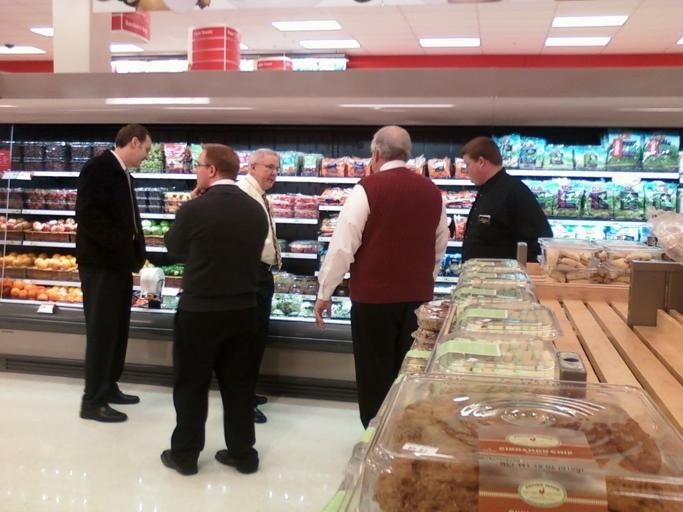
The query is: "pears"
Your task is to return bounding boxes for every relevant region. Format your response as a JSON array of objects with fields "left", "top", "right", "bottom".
[{"left": 0, "top": 252, "right": 78, "bottom": 270}]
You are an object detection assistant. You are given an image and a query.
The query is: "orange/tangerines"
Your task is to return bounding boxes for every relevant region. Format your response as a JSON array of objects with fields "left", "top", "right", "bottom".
[{"left": 0, "top": 279, "right": 83, "bottom": 303}]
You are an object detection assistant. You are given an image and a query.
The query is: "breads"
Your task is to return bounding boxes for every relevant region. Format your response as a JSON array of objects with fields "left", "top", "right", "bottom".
[{"left": 375, "top": 391, "right": 683, "bottom": 512}]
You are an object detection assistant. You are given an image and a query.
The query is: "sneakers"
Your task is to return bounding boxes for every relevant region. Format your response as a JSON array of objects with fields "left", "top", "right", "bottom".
[
  {"left": 254, "top": 396, "right": 268, "bottom": 424},
  {"left": 161, "top": 448, "right": 199, "bottom": 475},
  {"left": 216, "top": 448, "right": 259, "bottom": 473}
]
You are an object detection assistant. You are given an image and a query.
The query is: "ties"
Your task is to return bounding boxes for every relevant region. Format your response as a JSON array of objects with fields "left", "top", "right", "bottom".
[{"left": 262, "top": 194, "right": 282, "bottom": 270}]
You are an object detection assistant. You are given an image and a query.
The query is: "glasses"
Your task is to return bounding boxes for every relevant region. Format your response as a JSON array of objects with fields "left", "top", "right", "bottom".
[{"left": 193, "top": 160, "right": 210, "bottom": 169}]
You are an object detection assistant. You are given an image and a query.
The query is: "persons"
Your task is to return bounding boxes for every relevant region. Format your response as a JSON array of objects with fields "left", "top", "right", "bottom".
[
  {"left": 311, "top": 124, "right": 450, "bottom": 431},
  {"left": 459, "top": 136, "right": 553, "bottom": 263},
  {"left": 72, "top": 122, "right": 152, "bottom": 423},
  {"left": 235, "top": 147, "right": 281, "bottom": 424},
  {"left": 159, "top": 141, "right": 261, "bottom": 476}
]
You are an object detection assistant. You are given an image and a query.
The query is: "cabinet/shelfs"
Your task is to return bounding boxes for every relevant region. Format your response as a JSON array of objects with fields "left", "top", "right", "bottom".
[
  {"left": 0, "top": 120, "right": 680, "bottom": 404},
  {"left": 337, "top": 255, "right": 681, "bottom": 510}
]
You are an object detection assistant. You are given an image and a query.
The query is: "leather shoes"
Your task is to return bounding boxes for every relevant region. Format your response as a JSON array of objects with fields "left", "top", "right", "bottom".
[
  {"left": 79, "top": 397, "right": 126, "bottom": 422},
  {"left": 107, "top": 384, "right": 139, "bottom": 404}
]
